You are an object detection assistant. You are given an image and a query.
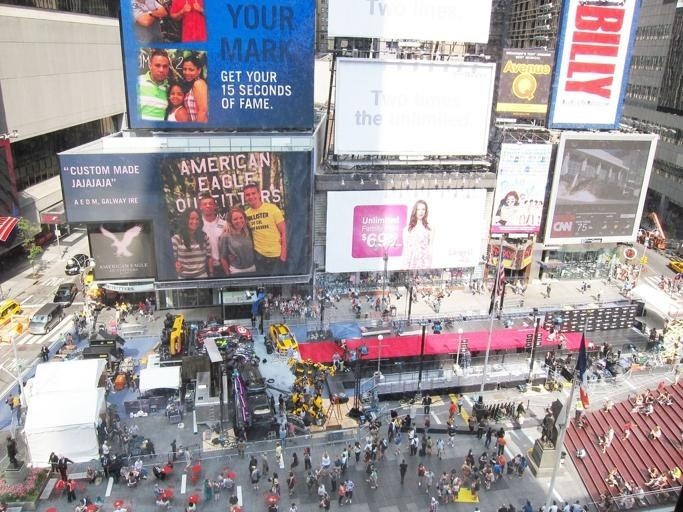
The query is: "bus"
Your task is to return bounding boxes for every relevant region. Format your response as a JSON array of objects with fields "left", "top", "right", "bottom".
[{"left": 0, "top": 298, "right": 20, "bottom": 329}]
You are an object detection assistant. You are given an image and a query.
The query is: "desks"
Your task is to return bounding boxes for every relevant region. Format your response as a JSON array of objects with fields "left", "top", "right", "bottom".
[{"left": 45, "top": 462, "right": 278, "bottom": 512}]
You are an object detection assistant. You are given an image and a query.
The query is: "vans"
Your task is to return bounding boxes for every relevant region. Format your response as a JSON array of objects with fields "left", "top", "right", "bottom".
[{"left": 29, "top": 302, "right": 66, "bottom": 336}]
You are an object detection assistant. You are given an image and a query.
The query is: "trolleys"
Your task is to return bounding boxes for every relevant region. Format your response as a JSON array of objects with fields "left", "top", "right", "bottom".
[{"left": 84, "top": 458, "right": 104, "bottom": 488}]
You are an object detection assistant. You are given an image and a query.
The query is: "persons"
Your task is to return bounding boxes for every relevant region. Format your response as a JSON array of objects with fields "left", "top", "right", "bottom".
[
  {"left": 58, "top": 301, "right": 97, "bottom": 347},
  {"left": 131, "top": 0, "right": 167, "bottom": 44},
  {"left": 258, "top": 259, "right": 642, "bottom": 322},
  {"left": 39, "top": 343, "right": 50, "bottom": 362},
  {"left": 46, "top": 392, "right": 532, "bottom": 511},
  {"left": 539, "top": 409, "right": 554, "bottom": 445},
  {"left": 169, "top": 208, "right": 211, "bottom": 280},
  {"left": 124, "top": 368, "right": 140, "bottom": 393},
  {"left": 4, "top": 393, "right": 14, "bottom": 411},
  {"left": 170, "top": 1, "right": 208, "bottom": 42},
  {"left": 242, "top": 183, "right": 288, "bottom": 277},
  {"left": 575, "top": 316, "right": 683, "bottom": 511},
  {"left": 199, "top": 194, "right": 229, "bottom": 278},
  {"left": 496, "top": 190, "right": 544, "bottom": 227},
  {"left": 399, "top": 198, "right": 435, "bottom": 268},
  {"left": 137, "top": 50, "right": 171, "bottom": 121},
  {"left": 331, "top": 350, "right": 342, "bottom": 366},
  {"left": 181, "top": 54, "right": 210, "bottom": 125},
  {"left": 12, "top": 394, "right": 19, "bottom": 410},
  {"left": 216, "top": 204, "right": 258, "bottom": 277},
  {"left": 114, "top": 295, "right": 156, "bottom": 324},
  {"left": 165, "top": 82, "right": 191, "bottom": 123},
  {"left": 538, "top": 498, "right": 582, "bottom": 511},
  {"left": 656, "top": 271, "right": 683, "bottom": 296},
  {"left": 5, "top": 436, "right": 19, "bottom": 468}
]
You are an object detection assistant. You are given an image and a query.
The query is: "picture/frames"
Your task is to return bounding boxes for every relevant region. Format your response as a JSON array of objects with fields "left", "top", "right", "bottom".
[{"left": 548, "top": 0, "right": 643, "bottom": 130}]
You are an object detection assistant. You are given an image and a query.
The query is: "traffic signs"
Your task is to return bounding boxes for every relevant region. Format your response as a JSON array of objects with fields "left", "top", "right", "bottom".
[{"left": 523, "top": 333, "right": 543, "bottom": 349}]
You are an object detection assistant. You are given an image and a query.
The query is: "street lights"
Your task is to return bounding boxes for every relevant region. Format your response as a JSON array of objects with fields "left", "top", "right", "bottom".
[
  {"left": 376, "top": 335, "right": 384, "bottom": 378},
  {"left": 471, "top": 235, "right": 530, "bottom": 423},
  {"left": 381, "top": 250, "right": 390, "bottom": 286},
  {"left": 415, "top": 317, "right": 426, "bottom": 392},
  {"left": 634, "top": 227, "right": 660, "bottom": 282},
  {"left": 316, "top": 266, "right": 328, "bottom": 326},
  {"left": 453, "top": 328, "right": 463, "bottom": 368},
  {"left": 348, "top": 342, "right": 370, "bottom": 423}
]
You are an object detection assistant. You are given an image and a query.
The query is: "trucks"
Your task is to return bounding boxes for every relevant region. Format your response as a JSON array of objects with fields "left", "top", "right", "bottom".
[{"left": 227, "top": 364, "right": 278, "bottom": 436}]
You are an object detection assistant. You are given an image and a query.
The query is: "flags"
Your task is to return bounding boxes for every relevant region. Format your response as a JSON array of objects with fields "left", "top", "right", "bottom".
[{"left": 496, "top": 252, "right": 508, "bottom": 297}]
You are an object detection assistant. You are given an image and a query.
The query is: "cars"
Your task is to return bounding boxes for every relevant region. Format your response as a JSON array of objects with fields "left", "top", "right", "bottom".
[
  {"left": 66, "top": 253, "right": 92, "bottom": 276},
  {"left": 155, "top": 312, "right": 305, "bottom": 362},
  {"left": 669, "top": 261, "right": 683, "bottom": 273},
  {"left": 52, "top": 284, "right": 79, "bottom": 308}
]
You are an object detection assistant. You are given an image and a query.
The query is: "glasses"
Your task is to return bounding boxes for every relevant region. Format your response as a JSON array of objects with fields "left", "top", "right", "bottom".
[{"left": 506, "top": 199, "right": 516, "bottom": 204}]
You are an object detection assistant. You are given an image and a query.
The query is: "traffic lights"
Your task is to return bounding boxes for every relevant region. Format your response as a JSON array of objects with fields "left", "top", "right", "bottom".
[
  {"left": 645, "top": 257, "right": 647, "bottom": 263},
  {"left": 639, "top": 257, "right": 642, "bottom": 264}
]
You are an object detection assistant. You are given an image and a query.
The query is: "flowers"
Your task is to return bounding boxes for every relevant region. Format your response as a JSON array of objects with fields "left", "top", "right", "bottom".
[{"left": 0, "top": 468, "right": 45, "bottom": 501}]
